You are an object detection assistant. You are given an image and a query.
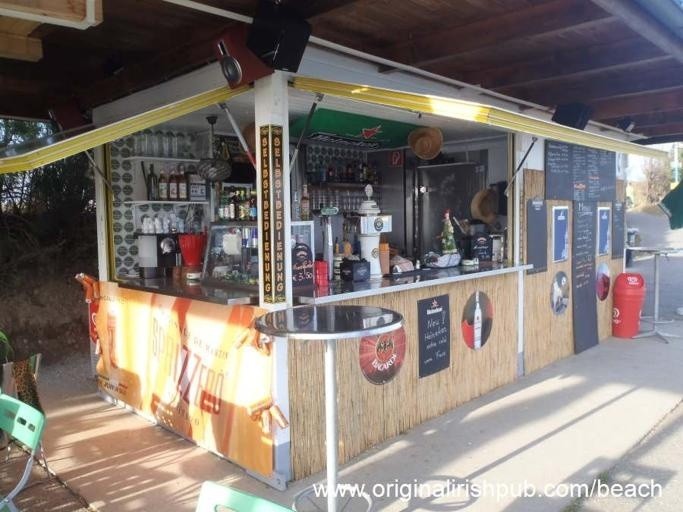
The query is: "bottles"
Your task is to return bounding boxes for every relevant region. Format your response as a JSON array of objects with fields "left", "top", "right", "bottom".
[
  {"left": 379, "top": 234, "right": 389, "bottom": 275},
  {"left": 496, "top": 236, "right": 504, "bottom": 264},
  {"left": 315, "top": 253, "right": 328, "bottom": 289},
  {"left": 290, "top": 160, "right": 381, "bottom": 222},
  {"left": 219, "top": 186, "right": 256, "bottom": 222},
  {"left": 132, "top": 129, "right": 201, "bottom": 201},
  {"left": 242, "top": 226, "right": 257, "bottom": 248}
]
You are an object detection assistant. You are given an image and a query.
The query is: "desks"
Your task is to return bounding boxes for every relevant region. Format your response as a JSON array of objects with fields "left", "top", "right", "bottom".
[
  {"left": 626, "top": 244, "right": 682, "bottom": 346},
  {"left": 252, "top": 305, "right": 404, "bottom": 512}
]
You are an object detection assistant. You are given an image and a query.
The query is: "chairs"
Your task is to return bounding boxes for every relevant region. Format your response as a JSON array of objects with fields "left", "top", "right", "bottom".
[{"left": 0, "top": 352, "right": 56, "bottom": 511}]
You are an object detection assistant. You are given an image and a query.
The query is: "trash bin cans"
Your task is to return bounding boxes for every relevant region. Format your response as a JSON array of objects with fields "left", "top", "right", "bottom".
[{"left": 612, "top": 272, "right": 646, "bottom": 337}]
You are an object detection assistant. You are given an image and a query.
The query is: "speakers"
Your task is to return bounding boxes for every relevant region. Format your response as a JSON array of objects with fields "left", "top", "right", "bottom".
[
  {"left": 247, "top": 9, "right": 312, "bottom": 72},
  {"left": 552, "top": 103, "right": 593, "bottom": 129},
  {"left": 216, "top": 27, "right": 274, "bottom": 90}
]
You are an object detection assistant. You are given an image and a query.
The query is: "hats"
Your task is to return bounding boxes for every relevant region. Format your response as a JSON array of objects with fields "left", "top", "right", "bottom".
[
  {"left": 468, "top": 187, "right": 500, "bottom": 225},
  {"left": 406, "top": 126, "right": 444, "bottom": 161}
]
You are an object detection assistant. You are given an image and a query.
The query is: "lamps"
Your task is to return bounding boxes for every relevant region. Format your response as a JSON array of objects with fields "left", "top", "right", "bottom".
[{"left": 198, "top": 115, "right": 232, "bottom": 189}]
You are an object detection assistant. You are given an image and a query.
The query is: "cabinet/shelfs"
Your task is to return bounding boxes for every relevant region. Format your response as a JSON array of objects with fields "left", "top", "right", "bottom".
[
  {"left": 121, "top": 153, "right": 209, "bottom": 238},
  {"left": 326, "top": 182, "right": 381, "bottom": 217}
]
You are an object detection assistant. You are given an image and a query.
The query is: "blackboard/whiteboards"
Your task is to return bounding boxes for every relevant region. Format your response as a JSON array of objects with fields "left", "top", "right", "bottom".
[{"left": 526, "top": 138, "right": 625, "bottom": 353}]
